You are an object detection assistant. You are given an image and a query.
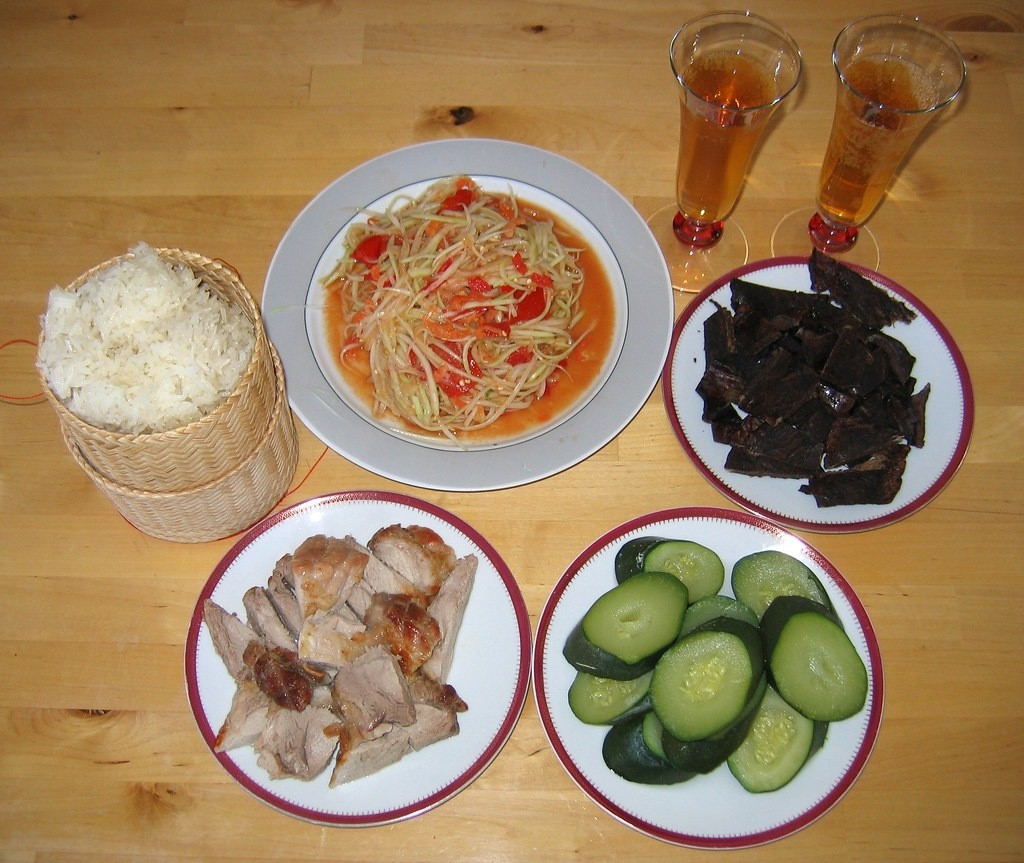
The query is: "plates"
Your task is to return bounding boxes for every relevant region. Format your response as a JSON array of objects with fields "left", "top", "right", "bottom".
[
  {"left": 260, "top": 137, "right": 675, "bottom": 494},
  {"left": 531, "top": 506, "right": 885, "bottom": 849},
  {"left": 662, "top": 254, "right": 975, "bottom": 535},
  {"left": 183, "top": 490, "right": 531, "bottom": 830}
]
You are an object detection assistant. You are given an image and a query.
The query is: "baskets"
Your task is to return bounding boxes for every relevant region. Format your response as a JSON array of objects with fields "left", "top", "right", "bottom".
[{"left": 35, "top": 247, "right": 301, "bottom": 544}]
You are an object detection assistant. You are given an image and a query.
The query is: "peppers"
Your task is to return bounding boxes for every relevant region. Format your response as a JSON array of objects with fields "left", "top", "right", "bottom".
[{"left": 350, "top": 180, "right": 550, "bottom": 407}]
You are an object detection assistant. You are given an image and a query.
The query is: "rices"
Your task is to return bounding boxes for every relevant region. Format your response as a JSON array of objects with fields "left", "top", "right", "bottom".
[{"left": 34, "top": 240, "right": 258, "bottom": 437}]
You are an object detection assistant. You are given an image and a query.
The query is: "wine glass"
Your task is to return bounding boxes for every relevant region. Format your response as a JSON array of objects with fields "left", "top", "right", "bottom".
[
  {"left": 644, "top": 10, "right": 802, "bottom": 295},
  {"left": 771, "top": 14, "right": 966, "bottom": 271}
]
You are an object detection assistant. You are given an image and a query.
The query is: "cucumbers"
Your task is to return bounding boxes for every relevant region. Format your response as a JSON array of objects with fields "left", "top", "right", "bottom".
[{"left": 564, "top": 537, "right": 868, "bottom": 794}]
